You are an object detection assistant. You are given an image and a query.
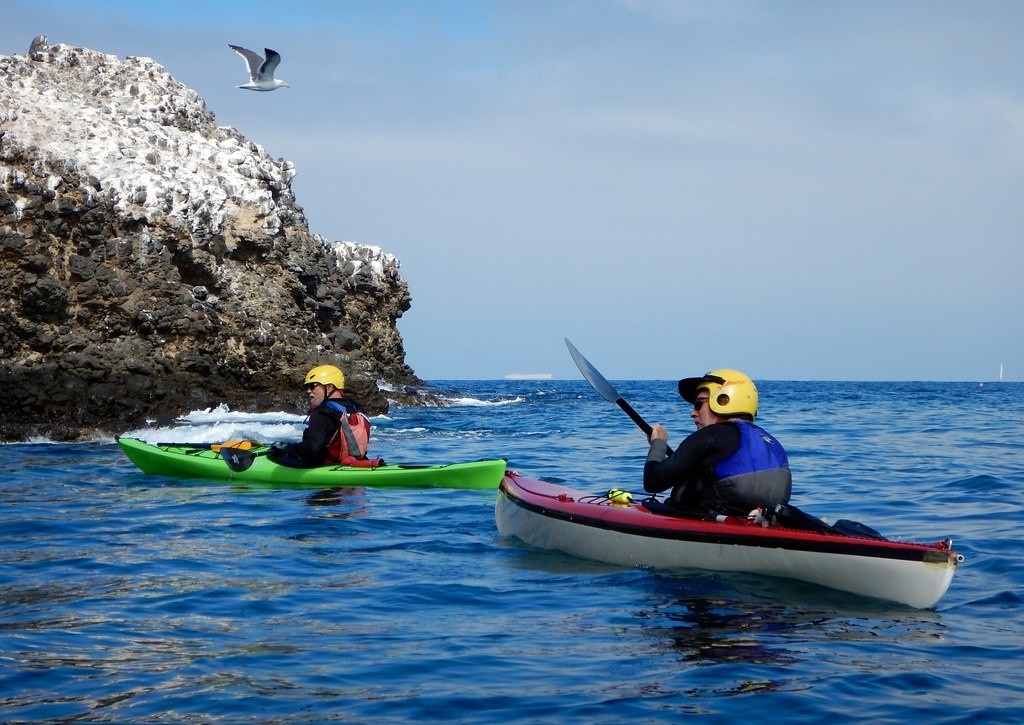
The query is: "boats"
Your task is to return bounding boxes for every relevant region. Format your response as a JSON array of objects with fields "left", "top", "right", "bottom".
[
  {"left": 495, "top": 470, "right": 965, "bottom": 608},
  {"left": 114, "top": 435, "right": 508, "bottom": 488}
]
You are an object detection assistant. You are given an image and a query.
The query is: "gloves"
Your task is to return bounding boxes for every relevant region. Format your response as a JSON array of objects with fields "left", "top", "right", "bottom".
[{"left": 270, "top": 440, "right": 290, "bottom": 454}]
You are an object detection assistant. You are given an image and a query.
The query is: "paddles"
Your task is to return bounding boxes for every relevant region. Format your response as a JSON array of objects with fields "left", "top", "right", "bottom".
[
  {"left": 218, "top": 446, "right": 276, "bottom": 473},
  {"left": 563, "top": 336, "right": 887, "bottom": 539}
]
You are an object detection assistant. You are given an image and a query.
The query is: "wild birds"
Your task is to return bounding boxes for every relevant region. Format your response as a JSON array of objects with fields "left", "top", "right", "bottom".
[{"left": 227, "top": 43, "right": 290, "bottom": 92}]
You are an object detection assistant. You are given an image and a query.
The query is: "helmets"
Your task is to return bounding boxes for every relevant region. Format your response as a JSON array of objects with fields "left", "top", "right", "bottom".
[
  {"left": 678, "top": 368, "right": 759, "bottom": 424},
  {"left": 304, "top": 364, "right": 345, "bottom": 390}
]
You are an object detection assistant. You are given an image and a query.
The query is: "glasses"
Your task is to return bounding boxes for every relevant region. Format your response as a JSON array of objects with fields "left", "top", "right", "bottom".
[
  {"left": 693, "top": 397, "right": 709, "bottom": 411},
  {"left": 305, "top": 382, "right": 321, "bottom": 391}
]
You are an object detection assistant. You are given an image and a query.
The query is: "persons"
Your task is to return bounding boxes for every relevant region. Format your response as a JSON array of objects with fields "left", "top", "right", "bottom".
[
  {"left": 640, "top": 368, "right": 789, "bottom": 517},
  {"left": 270, "top": 364, "right": 368, "bottom": 468}
]
